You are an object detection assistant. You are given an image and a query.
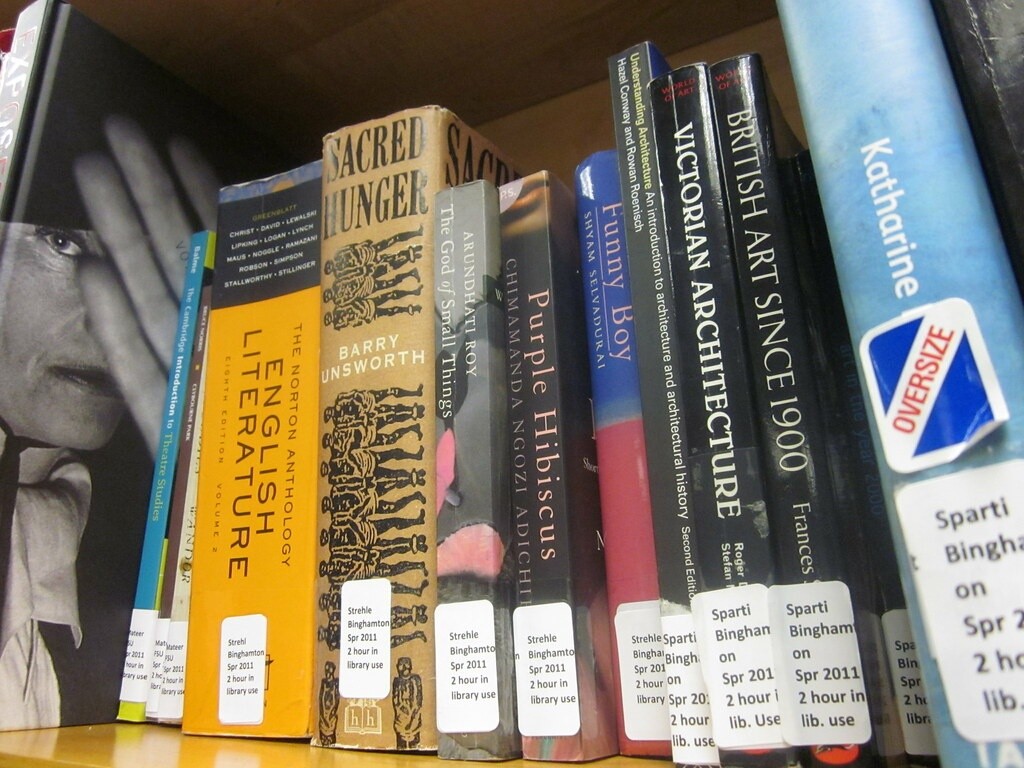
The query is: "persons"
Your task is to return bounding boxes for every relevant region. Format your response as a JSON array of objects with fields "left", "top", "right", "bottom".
[{"left": 0, "top": 115, "right": 232, "bottom": 731}]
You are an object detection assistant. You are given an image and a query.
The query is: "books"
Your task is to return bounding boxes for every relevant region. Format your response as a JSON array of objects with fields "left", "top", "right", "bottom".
[{"left": 0, "top": 0, "right": 1024, "bottom": 768}]
